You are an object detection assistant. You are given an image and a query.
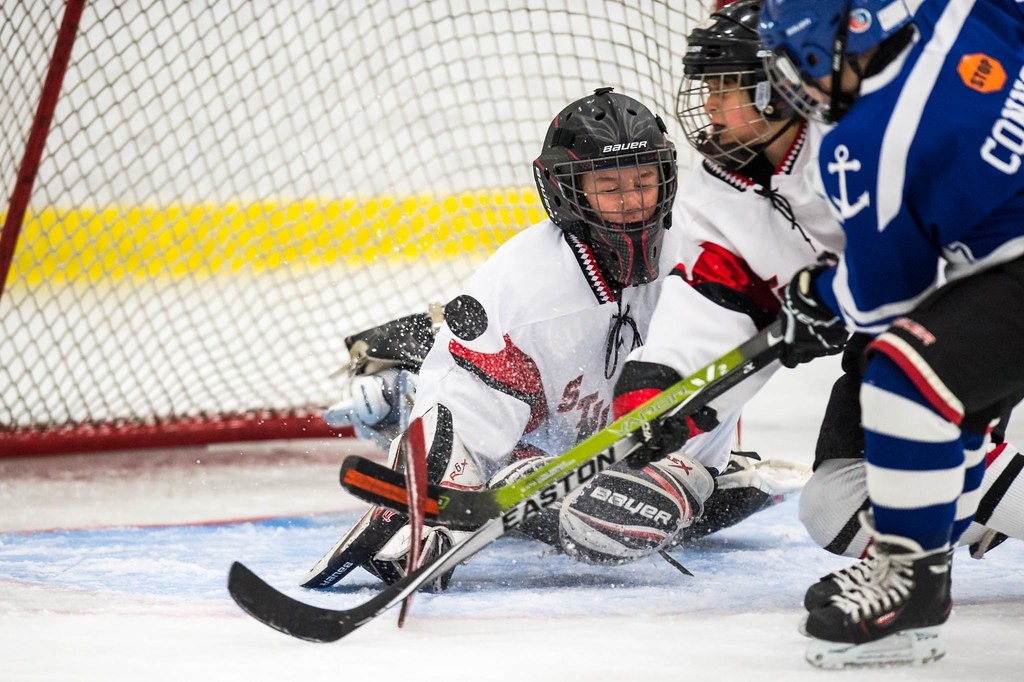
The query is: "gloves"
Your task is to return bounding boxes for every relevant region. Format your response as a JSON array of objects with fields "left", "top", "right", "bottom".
[
  {"left": 625, "top": 415, "right": 690, "bottom": 470},
  {"left": 778, "top": 251, "right": 850, "bottom": 368}
]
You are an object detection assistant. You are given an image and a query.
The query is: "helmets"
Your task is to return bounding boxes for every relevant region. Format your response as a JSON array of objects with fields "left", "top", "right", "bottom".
[
  {"left": 676, "top": 0, "right": 801, "bottom": 186},
  {"left": 533, "top": 87, "right": 678, "bottom": 287},
  {"left": 756, "top": 0, "right": 924, "bottom": 124}
]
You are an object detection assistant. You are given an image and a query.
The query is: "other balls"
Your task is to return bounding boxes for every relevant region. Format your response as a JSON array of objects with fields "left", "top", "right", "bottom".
[{"left": 446, "top": 295, "right": 488, "bottom": 341}]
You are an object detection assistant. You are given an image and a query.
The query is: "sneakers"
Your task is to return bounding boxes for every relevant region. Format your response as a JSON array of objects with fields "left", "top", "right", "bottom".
[{"left": 796, "top": 506, "right": 955, "bottom": 671}]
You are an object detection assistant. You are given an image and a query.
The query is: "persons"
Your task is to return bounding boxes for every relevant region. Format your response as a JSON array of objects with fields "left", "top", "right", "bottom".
[{"left": 303, "top": 0, "right": 1024, "bottom": 667}]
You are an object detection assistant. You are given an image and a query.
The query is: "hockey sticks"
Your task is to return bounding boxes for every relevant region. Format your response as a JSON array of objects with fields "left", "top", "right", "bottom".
[
  {"left": 227, "top": 339, "right": 784, "bottom": 644},
  {"left": 339, "top": 317, "right": 786, "bottom": 536}
]
